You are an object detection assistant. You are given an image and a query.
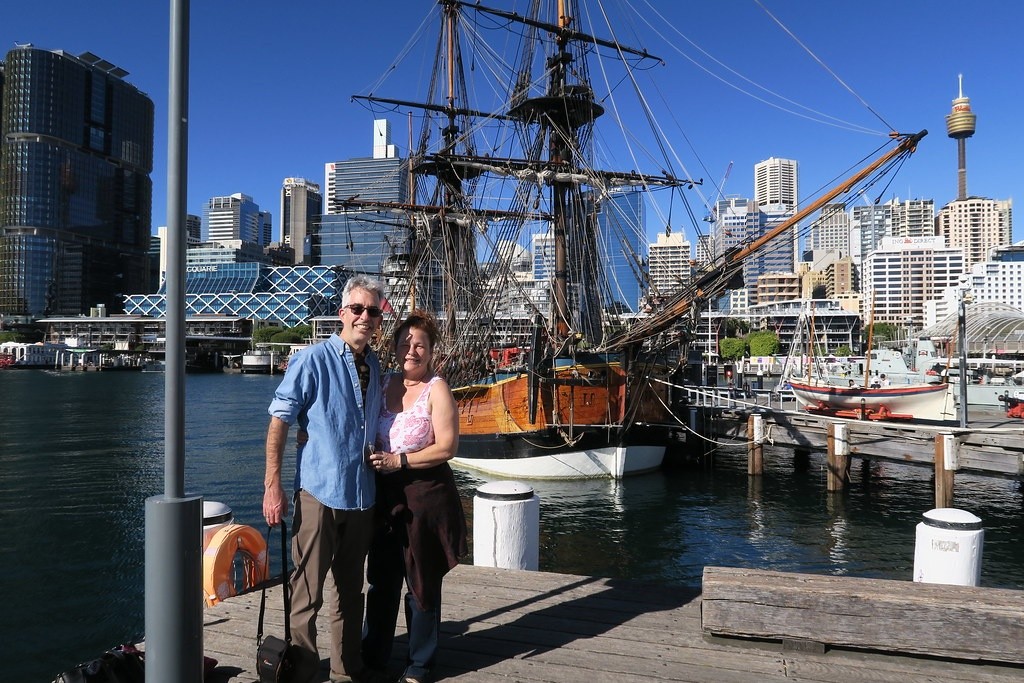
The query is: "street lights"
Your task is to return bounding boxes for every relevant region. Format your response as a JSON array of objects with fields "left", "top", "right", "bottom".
[{"left": 954, "top": 273, "right": 971, "bottom": 426}]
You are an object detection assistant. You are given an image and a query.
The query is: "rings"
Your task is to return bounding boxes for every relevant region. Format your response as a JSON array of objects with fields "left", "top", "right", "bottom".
[
  {"left": 377, "top": 460, "right": 379, "bottom": 465},
  {"left": 265, "top": 515, "right": 269, "bottom": 517}
]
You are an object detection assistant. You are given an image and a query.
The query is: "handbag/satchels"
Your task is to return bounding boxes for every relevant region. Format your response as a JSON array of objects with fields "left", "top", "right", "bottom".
[{"left": 255, "top": 520, "right": 315, "bottom": 683}]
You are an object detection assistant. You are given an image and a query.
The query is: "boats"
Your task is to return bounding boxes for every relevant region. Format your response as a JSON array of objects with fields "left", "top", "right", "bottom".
[
  {"left": 768, "top": 299, "right": 831, "bottom": 401},
  {"left": 947, "top": 368, "right": 1024, "bottom": 405},
  {"left": 241, "top": 334, "right": 285, "bottom": 374},
  {"left": 787, "top": 298, "right": 971, "bottom": 426},
  {"left": 275, "top": 342, "right": 311, "bottom": 374}
]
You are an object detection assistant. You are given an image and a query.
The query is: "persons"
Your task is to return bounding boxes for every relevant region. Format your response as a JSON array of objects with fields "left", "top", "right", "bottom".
[
  {"left": 849, "top": 379, "right": 858, "bottom": 388},
  {"left": 879, "top": 374, "right": 889, "bottom": 387},
  {"left": 296, "top": 310, "right": 469, "bottom": 683},
  {"left": 262, "top": 276, "right": 385, "bottom": 683}
]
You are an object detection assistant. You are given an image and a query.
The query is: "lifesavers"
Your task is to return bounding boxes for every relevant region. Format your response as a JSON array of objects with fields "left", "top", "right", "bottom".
[{"left": 205, "top": 524, "right": 269, "bottom": 608}]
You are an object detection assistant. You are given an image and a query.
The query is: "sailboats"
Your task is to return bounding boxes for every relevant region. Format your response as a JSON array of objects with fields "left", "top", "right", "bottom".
[{"left": 336, "top": 0, "right": 932, "bottom": 480}]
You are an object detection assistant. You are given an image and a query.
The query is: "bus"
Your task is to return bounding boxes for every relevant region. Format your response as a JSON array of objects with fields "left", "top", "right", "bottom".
[{"left": 941, "top": 369, "right": 973, "bottom": 385}]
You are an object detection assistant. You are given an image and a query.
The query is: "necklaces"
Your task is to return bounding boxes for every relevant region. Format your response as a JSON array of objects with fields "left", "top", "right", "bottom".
[{"left": 400, "top": 371, "right": 429, "bottom": 386}]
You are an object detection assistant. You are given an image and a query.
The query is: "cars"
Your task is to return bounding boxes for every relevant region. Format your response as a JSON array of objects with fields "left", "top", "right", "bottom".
[
  {"left": 991, "top": 376, "right": 1006, "bottom": 385},
  {"left": 922, "top": 370, "right": 943, "bottom": 384},
  {"left": 2, "top": 318, "right": 16, "bottom": 330}
]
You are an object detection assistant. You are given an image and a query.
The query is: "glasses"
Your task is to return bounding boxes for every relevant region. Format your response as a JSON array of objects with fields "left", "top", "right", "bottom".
[{"left": 343, "top": 302, "right": 381, "bottom": 318}]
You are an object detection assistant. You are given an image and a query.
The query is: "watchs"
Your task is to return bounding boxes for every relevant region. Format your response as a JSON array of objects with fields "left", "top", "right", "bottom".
[{"left": 400, "top": 453, "right": 407, "bottom": 470}]
